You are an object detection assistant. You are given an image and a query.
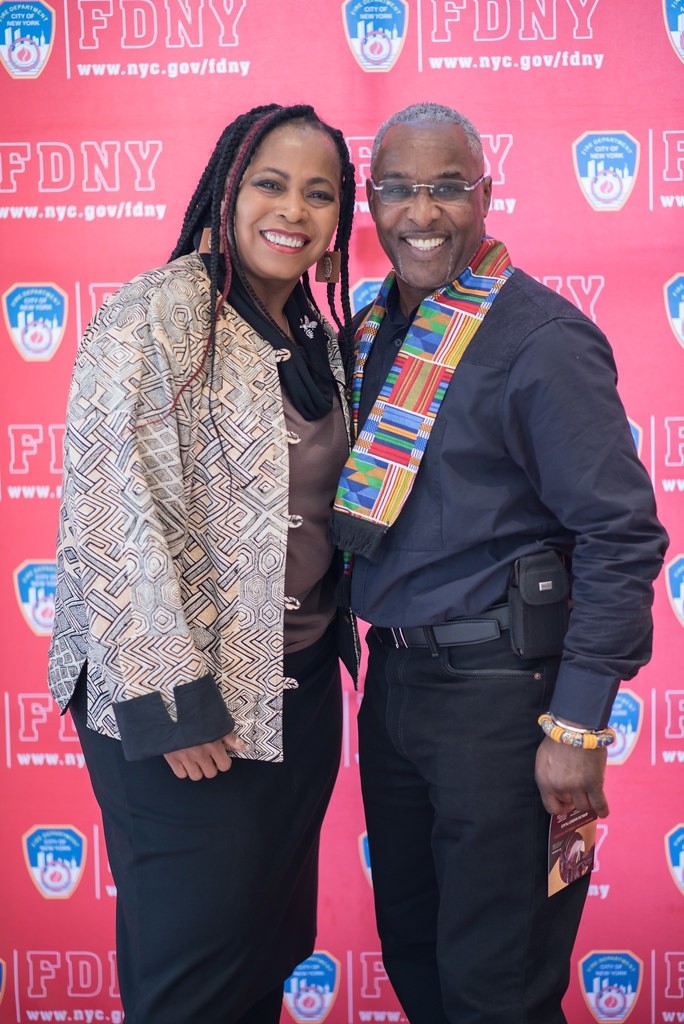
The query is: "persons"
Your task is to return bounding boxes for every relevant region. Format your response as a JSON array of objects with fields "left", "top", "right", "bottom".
[
  {"left": 48, "top": 103, "right": 361, "bottom": 1024},
  {"left": 330, "top": 102, "right": 669, "bottom": 1024}
]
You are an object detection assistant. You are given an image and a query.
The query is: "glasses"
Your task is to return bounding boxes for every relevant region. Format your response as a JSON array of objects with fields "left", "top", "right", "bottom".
[{"left": 370, "top": 177, "right": 483, "bottom": 206}]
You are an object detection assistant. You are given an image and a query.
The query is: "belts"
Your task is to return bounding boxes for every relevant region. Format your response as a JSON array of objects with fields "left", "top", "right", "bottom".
[{"left": 373, "top": 605, "right": 509, "bottom": 650}]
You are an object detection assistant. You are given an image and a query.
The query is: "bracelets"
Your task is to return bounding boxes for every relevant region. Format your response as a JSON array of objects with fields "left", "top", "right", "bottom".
[{"left": 538, "top": 711, "right": 617, "bottom": 749}]
